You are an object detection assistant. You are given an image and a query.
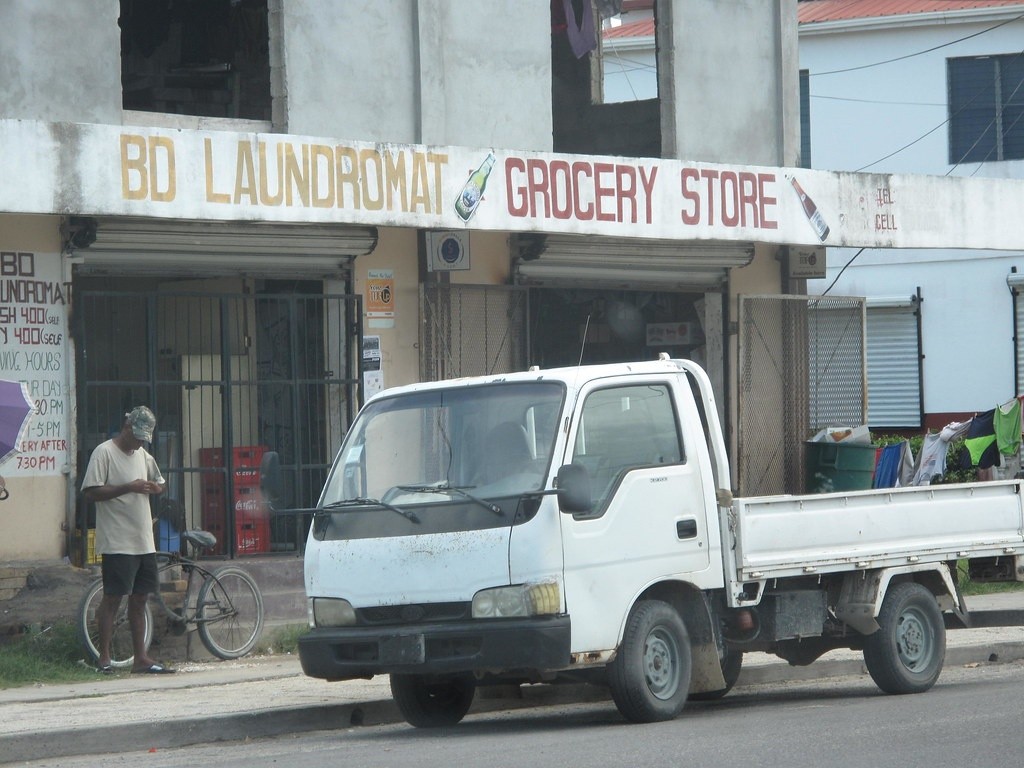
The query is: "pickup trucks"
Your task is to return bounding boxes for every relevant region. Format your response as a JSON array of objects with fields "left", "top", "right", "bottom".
[{"left": 258, "top": 351, "right": 1024, "bottom": 728}]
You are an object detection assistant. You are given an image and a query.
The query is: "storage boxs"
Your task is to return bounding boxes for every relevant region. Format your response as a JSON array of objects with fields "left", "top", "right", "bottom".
[
  {"left": 74, "top": 526, "right": 103, "bottom": 567},
  {"left": 195, "top": 445, "right": 271, "bottom": 559},
  {"left": 151, "top": 518, "right": 180, "bottom": 554}
]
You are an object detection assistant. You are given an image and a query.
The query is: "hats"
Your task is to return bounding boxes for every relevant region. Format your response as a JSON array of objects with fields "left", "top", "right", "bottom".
[{"left": 128, "top": 406, "right": 157, "bottom": 445}]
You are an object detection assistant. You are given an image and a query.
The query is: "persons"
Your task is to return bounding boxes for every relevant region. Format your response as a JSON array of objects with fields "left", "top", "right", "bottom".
[
  {"left": 80, "top": 406, "right": 174, "bottom": 673},
  {"left": 0, "top": 476, "right": 5, "bottom": 496}
]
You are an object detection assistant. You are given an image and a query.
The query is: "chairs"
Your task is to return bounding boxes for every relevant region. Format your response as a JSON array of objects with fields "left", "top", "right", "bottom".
[{"left": 484, "top": 420, "right": 536, "bottom": 489}]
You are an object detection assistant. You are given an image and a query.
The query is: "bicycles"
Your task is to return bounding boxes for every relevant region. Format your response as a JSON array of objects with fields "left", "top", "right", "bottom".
[{"left": 78, "top": 506, "right": 266, "bottom": 671}]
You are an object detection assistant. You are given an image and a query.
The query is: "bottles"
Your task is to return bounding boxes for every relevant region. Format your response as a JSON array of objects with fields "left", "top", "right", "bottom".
[{"left": 455, "top": 152, "right": 495, "bottom": 221}]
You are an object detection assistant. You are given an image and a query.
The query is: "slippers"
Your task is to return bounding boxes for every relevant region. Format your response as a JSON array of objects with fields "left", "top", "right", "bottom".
[
  {"left": 100, "top": 665, "right": 115, "bottom": 675},
  {"left": 130, "top": 663, "right": 177, "bottom": 673}
]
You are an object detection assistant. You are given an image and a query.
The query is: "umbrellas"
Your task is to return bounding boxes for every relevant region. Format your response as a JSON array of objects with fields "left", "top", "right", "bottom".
[{"left": 0, "top": 379, "right": 36, "bottom": 499}]
